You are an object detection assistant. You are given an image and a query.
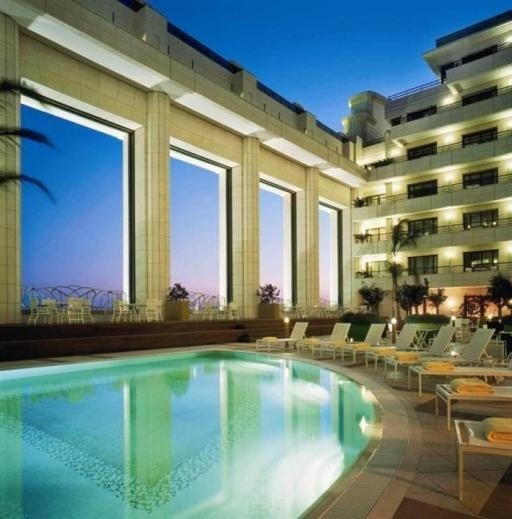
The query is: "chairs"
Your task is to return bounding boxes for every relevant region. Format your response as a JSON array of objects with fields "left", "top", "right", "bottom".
[
  {"left": 285, "top": 305, "right": 343, "bottom": 317},
  {"left": 190, "top": 301, "right": 240, "bottom": 321},
  {"left": 434, "top": 384, "right": 512, "bottom": 429},
  {"left": 256, "top": 321, "right": 385, "bottom": 364},
  {"left": 109, "top": 297, "right": 165, "bottom": 324},
  {"left": 407, "top": 354, "right": 512, "bottom": 397},
  {"left": 455, "top": 416, "right": 512, "bottom": 502},
  {"left": 28, "top": 297, "right": 95, "bottom": 326},
  {"left": 363, "top": 323, "right": 496, "bottom": 381}
]
